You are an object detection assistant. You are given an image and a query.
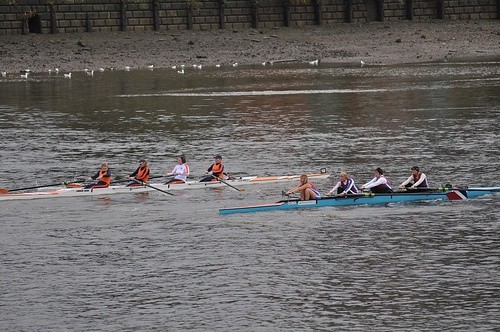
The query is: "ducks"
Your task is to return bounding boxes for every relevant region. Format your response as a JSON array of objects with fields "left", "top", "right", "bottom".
[
  {"left": 360, "top": 60, "right": 364, "bottom": 64},
  {"left": 172, "top": 66, "right": 176, "bottom": 68},
  {"left": 20, "top": 73, "right": 29, "bottom": 78},
  {"left": 84, "top": 68, "right": 105, "bottom": 75},
  {"left": 233, "top": 63, "right": 238, "bottom": 67},
  {"left": 178, "top": 69, "right": 184, "bottom": 74},
  {"left": 48, "top": 68, "right": 59, "bottom": 72},
  {"left": 215, "top": 64, "right": 220, "bottom": 67},
  {"left": 262, "top": 62, "right": 265, "bottom": 66},
  {"left": 148, "top": 65, "right": 153, "bottom": 68},
  {"left": 125, "top": 66, "right": 130, "bottom": 69},
  {"left": 64, "top": 72, "right": 72, "bottom": 76},
  {"left": 181, "top": 64, "right": 184, "bottom": 67},
  {"left": 308, "top": 59, "right": 318, "bottom": 65},
  {"left": 193, "top": 64, "right": 202, "bottom": 69}
]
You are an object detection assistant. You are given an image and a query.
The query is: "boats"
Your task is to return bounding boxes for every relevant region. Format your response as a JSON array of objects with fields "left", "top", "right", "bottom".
[
  {"left": 0, "top": 167, "right": 334, "bottom": 201},
  {"left": 218, "top": 182, "right": 500, "bottom": 215}
]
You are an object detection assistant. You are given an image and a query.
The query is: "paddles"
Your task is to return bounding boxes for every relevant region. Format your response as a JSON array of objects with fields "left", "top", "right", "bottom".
[
  {"left": 129, "top": 177, "right": 177, "bottom": 197},
  {"left": 282, "top": 190, "right": 301, "bottom": 199},
  {"left": 84, "top": 174, "right": 178, "bottom": 186},
  {"left": 398, "top": 186, "right": 500, "bottom": 194},
  {"left": 0, "top": 181, "right": 87, "bottom": 192},
  {"left": 206, "top": 171, "right": 245, "bottom": 193},
  {"left": 318, "top": 187, "right": 469, "bottom": 200}
]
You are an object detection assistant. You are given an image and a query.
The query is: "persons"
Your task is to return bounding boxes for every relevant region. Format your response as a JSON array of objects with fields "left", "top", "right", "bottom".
[
  {"left": 286, "top": 175, "right": 321, "bottom": 201},
  {"left": 328, "top": 171, "right": 359, "bottom": 196},
  {"left": 164, "top": 154, "right": 189, "bottom": 184},
  {"left": 200, "top": 154, "right": 224, "bottom": 182},
  {"left": 126, "top": 159, "right": 150, "bottom": 186},
  {"left": 361, "top": 167, "right": 394, "bottom": 193},
  {"left": 400, "top": 165, "right": 428, "bottom": 191},
  {"left": 84, "top": 161, "right": 111, "bottom": 192}
]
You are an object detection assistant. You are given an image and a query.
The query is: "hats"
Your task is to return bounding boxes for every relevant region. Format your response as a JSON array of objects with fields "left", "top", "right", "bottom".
[
  {"left": 411, "top": 166, "right": 419, "bottom": 173},
  {"left": 139, "top": 159, "right": 144, "bottom": 162},
  {"left": 213, "top": 154, "right": 222, "bottom": 158}
]
